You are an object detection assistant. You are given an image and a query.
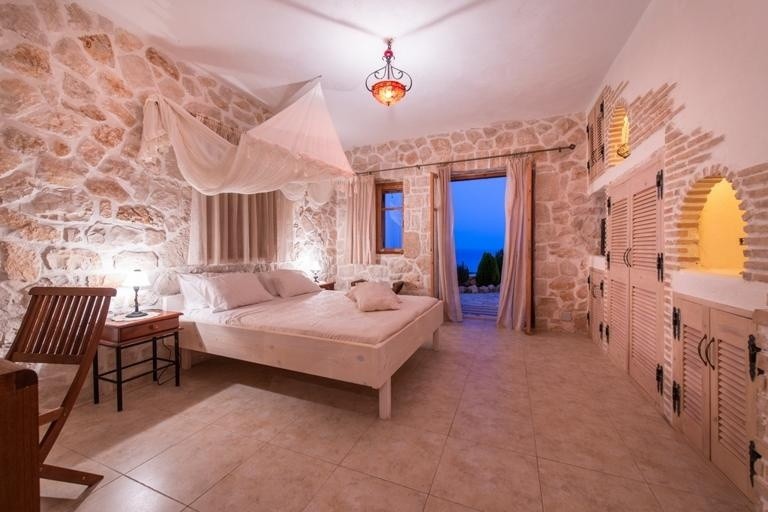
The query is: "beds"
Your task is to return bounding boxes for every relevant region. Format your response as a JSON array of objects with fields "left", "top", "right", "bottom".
[{"left": 163, "top": 290, "right": 444, "bottom": 421}]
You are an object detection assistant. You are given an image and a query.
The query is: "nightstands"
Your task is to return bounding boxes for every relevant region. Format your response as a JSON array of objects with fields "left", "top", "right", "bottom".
[
  {"left": 94, "top": 308, "right": 183, "bottom": 411},
  {"left": 320, "top": 281, "right": 336, "bottom": 290}
]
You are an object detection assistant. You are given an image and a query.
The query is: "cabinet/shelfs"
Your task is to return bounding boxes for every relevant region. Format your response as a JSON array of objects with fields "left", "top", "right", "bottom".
[
  {"left": 0, "top": 357, "right": 40, "bottom": 512},
  {"left": 671, "top": 295, "right": 754, "bottom": 503},
  {"left": 605, "top": 156, "right": 665, "bottom": 412},
  {"left": 586, "top": 92, "right": 605, "bottom": 182},
  {"left": 586, "top": 270, "right": 606, "bottom": 352}
]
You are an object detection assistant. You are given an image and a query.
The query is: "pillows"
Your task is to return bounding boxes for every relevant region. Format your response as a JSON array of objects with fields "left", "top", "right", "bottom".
[
  {"left": 346, "top": 282, "right": 400, "bottom": 313},
  {"left": 184, "top": 272, "right": 272, "bottom": 312},
  {"left": 272, "top": 273, "right": 322, "bottom": 298}
]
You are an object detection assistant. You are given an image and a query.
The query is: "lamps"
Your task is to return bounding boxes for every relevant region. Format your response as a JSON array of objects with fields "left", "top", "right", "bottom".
[
  {"left": 366, "top": 39, "right": 412, "bottom": 108},
  {"left": 120, "top": 268, "right": 152, "bottom": 318},
  {"left": 310, "top": 262, "right": 321, "bottom": 283}
]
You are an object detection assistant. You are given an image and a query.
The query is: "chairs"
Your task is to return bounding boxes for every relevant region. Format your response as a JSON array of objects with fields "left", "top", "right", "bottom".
[{"left": 6, "top": 286, "right": 117, "bottom": 488}]
